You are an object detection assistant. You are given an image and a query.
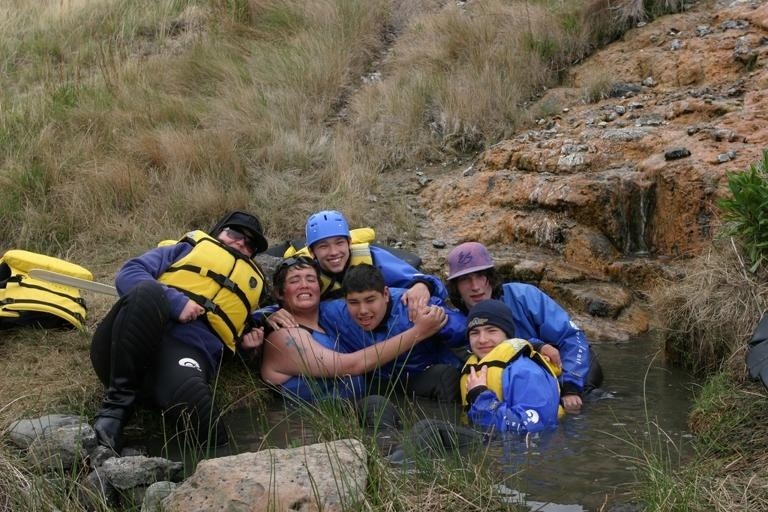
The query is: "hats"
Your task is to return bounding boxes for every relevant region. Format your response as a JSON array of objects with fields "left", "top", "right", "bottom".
[
  {"left": 221, "top": 211, "right": 268, "bottom": 253},
  {"left": 464, "top": 299, "right": 516, "bottom": 340}
]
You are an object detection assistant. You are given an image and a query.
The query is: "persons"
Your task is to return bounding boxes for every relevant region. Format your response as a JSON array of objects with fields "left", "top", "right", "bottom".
[
  {"left": 445, "top": 242, "right": 604, "bottom": 410},
  {"left": 385, "top": 299, "right": 565, "bottom": 470},
  {"left": 85, "top": 210, "right": 269, "bottom": 460},
  {"left": 252, "top": 207, "right": 470, "bottom": 454}
]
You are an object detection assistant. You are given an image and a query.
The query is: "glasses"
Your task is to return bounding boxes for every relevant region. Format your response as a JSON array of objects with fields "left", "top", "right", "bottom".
[
  {"left": 277, "top": 256, "right": 320, "bottom": 274},
  {"left": 221, "top": 226, "right": 259, "bottom": 254}
]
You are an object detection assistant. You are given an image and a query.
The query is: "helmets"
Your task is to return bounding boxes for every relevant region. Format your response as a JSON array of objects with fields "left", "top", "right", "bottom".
[
  {"left": 445, "top": 242, "right": 493, "bottom": 282},
  {"left": 304, "top": 209, "right": 352, "bottom": 247}
]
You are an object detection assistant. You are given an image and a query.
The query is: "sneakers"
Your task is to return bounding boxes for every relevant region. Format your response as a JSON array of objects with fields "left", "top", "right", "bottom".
[{"left": 94, "top": 417, "right": 123, "bottom": 448}]
